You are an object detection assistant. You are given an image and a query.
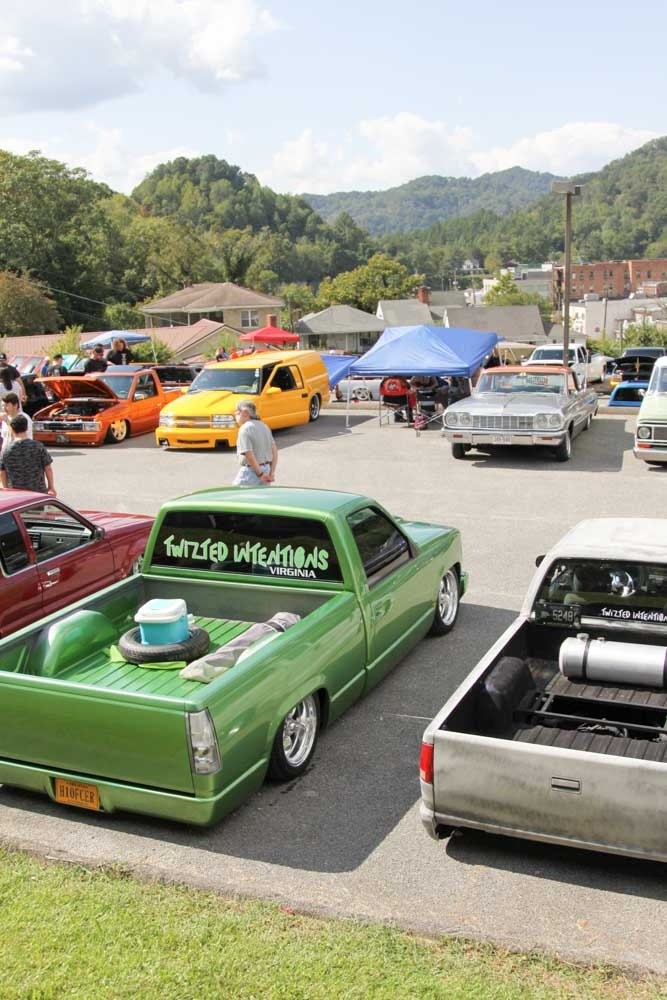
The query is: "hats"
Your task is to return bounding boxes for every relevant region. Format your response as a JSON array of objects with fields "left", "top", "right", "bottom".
[
  {"left": 0, "top": 353, "right": 6, "bottom": 360},
  {"left": 44, "top": 356, "right": 51, "bottom": 360},
  {"left": 53, "top": 354, "right": 63, "bottom": 358},
  {"left": 237, "top": 399, "right": 259, "bottom": 419}
]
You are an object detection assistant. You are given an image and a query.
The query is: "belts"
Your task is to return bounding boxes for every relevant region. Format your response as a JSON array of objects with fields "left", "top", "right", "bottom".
[{"left": 243, "top": 462, "right": 270, "bottom": 467}]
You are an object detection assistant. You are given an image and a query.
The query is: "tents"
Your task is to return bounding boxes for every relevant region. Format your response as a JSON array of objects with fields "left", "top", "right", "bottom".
[
  {"left": 346, "top": 326, "right": 505, "bottom": 428},
  {"left": 239, "top": 325, "right": 300, "bottom": 350},
  {"left": 80, "top": 331, "right": 157, "bottom": 363},
  {"left": 320, "top": 354, "right": 359, "bottom": 391}
]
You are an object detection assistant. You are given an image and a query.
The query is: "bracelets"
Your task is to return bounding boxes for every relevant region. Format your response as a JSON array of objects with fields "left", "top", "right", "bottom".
[{"left": 256, "top": 471, "right": 264, "bottom": 477}]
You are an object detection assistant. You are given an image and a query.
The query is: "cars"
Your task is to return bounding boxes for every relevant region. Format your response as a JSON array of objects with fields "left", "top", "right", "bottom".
[
  {"left": 0, "top": 487, "right": 156, "bottom": 642},
  {"left": 153, "top": 347, "right": 331, "bottom": 453},
  {"left": 2, "top": 353, "right": 204, "bottom": 449},
  {"left": 633, "top": 355, "right": 667, "bottom": 466},
  {"left": 439, "top": 362, "right": 597, "bottom": 461},
  {"left": 600, "top": 347, "right": 667, "bottom": 383},
  {"left": 526, "top": 344, "right": 591, "bottom": 389}
]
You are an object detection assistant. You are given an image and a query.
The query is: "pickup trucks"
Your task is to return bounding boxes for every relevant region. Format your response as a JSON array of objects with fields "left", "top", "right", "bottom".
[
  {"left": 0, "top": 484, "right": 471, "bottom": 832},
  {"left": 415, "top": 518, "right": 667, "bottom": 877}
]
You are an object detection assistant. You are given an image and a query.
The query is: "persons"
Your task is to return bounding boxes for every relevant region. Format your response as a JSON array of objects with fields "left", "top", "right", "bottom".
[
  {"left": 232, "top": 400, "right": 277, "bottom": 485},
  {"left": 484, "top": 348, "right": 512, "bottom": 369},
  {"left": 575, "top": 566, "right": 614, "bottom": 593},
  {"left": 382, "top": 375, "right": 464, "bottom": 423},
  {"left": 0, "top": 338, "right": 135, "bottom": 511},
  {"left": 215, "top": 345, "right": 255, "bottom": 363}
]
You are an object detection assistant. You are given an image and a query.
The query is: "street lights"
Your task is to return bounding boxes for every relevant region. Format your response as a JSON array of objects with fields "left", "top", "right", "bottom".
[{"left": 551, "top": 179, "right": 586, "bottom": 363}]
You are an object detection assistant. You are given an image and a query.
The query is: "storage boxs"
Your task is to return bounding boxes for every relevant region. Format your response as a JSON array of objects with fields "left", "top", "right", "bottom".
[{"left": 135, "top": 597, "right": 191, "bottom": 646}]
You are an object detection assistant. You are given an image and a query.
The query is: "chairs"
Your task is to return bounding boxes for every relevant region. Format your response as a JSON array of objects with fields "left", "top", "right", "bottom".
[{"left": 565, "top": 566, "right": 603, "bottom": 592}]
